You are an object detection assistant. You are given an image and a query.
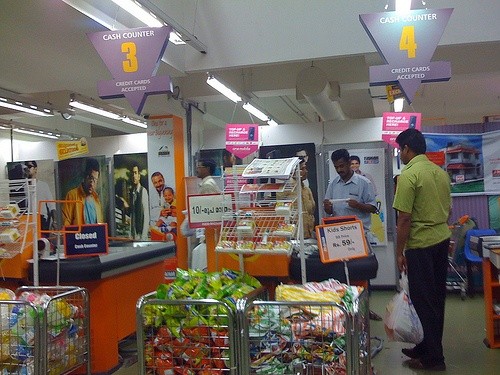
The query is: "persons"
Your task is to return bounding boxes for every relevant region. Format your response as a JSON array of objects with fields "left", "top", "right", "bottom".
[
  {"left": 149, "top": 172, "right": 176, "bottom": 235},
  {"left": 276, "top": 157, "right": 314, "bottom": 239},
  {"left": 195, "top": 159, "right": 220, "bottom": 194},
  {"left": 62, "top": 159, "right": 104, "bottom": 225},
  {"left": 129, "top": 165, "right": 149, "bottom": 239},
  {"left": 324, "top": 149, "right": 382, "bottom": 321},
  {"left": 392, "top": 129, "right": 452, "bottom": 371},
  {"left": 350, "top": 155, "right": 378, "bottom": 189},
  {"left": 24, "top": 161, "right": 56, "bottom": 238}
]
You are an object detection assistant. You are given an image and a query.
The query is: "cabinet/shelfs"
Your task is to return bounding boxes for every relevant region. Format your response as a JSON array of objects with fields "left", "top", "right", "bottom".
[
  {"left": 214, "top": 156, "right": 307, "bottom": 287},
  {"left": 482, "top": 258, "right": 500, "bottom": 349},
  {"left": 0, "top": 179, "right": 38, "bottom": 288}
]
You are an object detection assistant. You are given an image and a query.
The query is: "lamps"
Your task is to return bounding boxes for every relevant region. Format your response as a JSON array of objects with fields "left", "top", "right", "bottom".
[
  {"left": 0, "top": 87, "right": 148, "bottom": 140},
  {"left": 205, "top": 72, "right": 282, "bottom": 126},
  {"left": 113, "top": 0, "right": 187, "bottom": 46}
]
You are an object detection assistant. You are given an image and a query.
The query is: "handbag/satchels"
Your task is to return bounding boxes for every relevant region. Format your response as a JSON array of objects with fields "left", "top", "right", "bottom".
[{"left": 384, "top": 268, "right": 424, "bottom": 344}]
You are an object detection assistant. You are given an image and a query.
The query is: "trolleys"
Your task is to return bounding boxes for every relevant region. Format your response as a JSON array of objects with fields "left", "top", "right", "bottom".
[{"left": 445, "top": 255, "right": 473, "bottom": 296}]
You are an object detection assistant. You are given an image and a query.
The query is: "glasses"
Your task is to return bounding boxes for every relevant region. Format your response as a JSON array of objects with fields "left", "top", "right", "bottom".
[{"left": 28, "top": 165, "right": 35, "bottom": 168}]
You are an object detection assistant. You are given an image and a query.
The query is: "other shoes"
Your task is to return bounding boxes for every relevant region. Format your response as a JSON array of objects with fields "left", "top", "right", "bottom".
[
  {"left": 401, "top": 348, "right": 444, "bottom": 359},
  {"left": 406, "top": 358, "right": 446, "bottom": 370}
]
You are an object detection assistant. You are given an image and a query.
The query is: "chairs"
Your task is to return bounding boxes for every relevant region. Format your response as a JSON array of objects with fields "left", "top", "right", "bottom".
[{"left": 464, "top": 229, "right": 498, "bottom": 296}]
